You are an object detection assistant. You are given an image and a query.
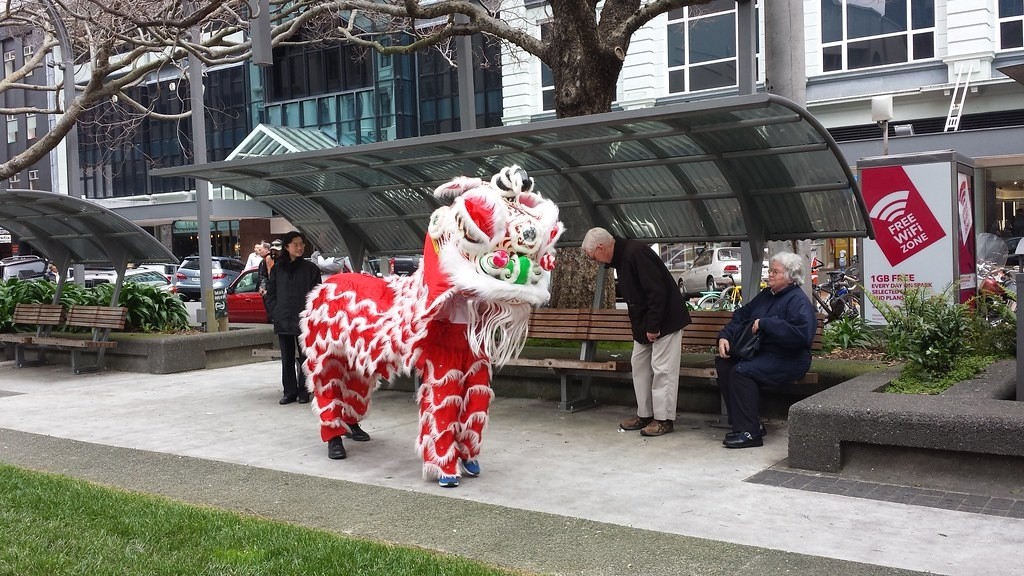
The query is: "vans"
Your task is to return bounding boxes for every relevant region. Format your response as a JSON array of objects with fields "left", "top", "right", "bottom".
[{"left": 137, "top": 263, "right": 180, "bottom": 281}]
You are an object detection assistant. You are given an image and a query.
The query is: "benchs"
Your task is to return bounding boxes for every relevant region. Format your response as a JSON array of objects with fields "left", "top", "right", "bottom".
[
  {"left": 487, "top": 308, "right": 824, "bottom": 429},
  {"left": 0, "top": 303, "right": 129, "bottom": 375}
]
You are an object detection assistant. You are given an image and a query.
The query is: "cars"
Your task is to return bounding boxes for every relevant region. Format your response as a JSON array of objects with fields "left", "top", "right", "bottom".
[
  {"left": 663, "top": 247, "right": 706, "bottom": 285},
  {"left": 677, "top": 246, "right": 771, "bottom": 301},
  {"left": 817, "top": 262, "right": 860, "bottom": 297},
  {"left": 225, "top": 264, "right": 352, "bottom": 323},
  {"left": 987, "top": 236, "right": 1023, "bottom": 265}
]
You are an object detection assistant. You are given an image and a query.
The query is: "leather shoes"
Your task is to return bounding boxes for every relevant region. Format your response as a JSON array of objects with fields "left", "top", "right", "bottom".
[
  {"left": 723, "top": 431, "right": 763, "bottom": 448},
  {"left": 725, "top": 424, "right": 767, "bottom": 440}
]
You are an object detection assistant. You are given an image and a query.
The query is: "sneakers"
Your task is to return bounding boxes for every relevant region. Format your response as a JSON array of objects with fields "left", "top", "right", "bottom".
[
  {"left": 460, "top": 456, "right": 480, "bottom": 477},
  {"left": 619, "top": 414, "right": 653, "bottom": 430},
  {"left": 345, "top": 423, "right": 370, "bottom": 441},
  {"left": 328, "top": 437, "right": 345, "bottom": 459},
  {"left": 438, "top": 475, "right": 459, "bottom": 487},
  {"left": 640, "top": 420, "right": 674, "bottom": 436}
]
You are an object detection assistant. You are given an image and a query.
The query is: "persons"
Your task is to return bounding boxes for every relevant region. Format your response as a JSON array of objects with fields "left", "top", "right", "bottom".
[
  {"left": 44, "top": 265, "right": 59, "bottom": 284},
  {"left": 244, "top": 243, "right": 263, "bottom": 271},
  {"left": 582, "top": 227, "right": 692, "bottom": 436},
  {"left": 258, "top": 243, "right": 274, "bottom": 324},
  {"left": 715, "top": 251, "right": 818, "bottom": 448},
  {"left": 265, "top": 231, "right": 323, "bottom": 404},
  {"left": 1011, "top": 209, "right": 1024, "bottom": 237}
]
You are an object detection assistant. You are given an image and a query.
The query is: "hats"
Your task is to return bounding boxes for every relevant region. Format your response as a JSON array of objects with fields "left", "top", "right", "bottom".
[{"left": 270, "top": 238, "right": 283, "bottom": 251}]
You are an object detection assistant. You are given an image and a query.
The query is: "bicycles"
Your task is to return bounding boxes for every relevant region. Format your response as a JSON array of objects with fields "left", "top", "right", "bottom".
[{"left": 698, "top": 271, "right": 862, "bottom": 324}]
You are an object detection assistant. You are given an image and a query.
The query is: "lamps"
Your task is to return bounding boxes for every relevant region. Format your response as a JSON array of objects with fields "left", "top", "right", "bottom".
[{"left": 894, "top": 124, "right": 914, "bottom": 136}]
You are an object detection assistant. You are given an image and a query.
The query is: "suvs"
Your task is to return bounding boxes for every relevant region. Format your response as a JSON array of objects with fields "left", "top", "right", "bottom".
[
  {"left": 173, "top": 256, "right": 245, "bottom": 302},
  {"left": 0, "top": 255, "right": 55, "bottom": 286},
  {"left": 368, "top": 255, "right": 419, "bottom": 276},
  {"left": 66, "top": 267, "right": 177, "bottom": 300}
]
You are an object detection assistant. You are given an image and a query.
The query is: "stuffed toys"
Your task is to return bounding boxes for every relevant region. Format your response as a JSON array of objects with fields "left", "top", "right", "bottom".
[{"left": 297, "top": 163, "right": 567, "bottom": 487}]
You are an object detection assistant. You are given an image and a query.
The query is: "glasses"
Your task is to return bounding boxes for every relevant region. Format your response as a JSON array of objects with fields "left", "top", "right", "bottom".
[
  {"left": 271, "top": 243, "right": 282, "bottom": 247},
  {"left": 768, "top": 270, "right": 784, "bottom": 275},
  {"left": 587, "top": 244, "right": 601, "bottom": 261}
]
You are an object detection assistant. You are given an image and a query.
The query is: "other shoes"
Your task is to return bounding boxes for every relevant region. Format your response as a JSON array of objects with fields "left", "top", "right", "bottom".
[
  {"left": 299, "top": 397, "right": 310, "bottom": 402},
  {"left": 279, "top": 398, "right": 296, "bottom": 404}
]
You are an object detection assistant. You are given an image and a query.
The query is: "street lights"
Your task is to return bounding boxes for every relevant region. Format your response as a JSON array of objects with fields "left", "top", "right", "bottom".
[{"left": 871, "top": 95, "right": 893, "bottom": 156}]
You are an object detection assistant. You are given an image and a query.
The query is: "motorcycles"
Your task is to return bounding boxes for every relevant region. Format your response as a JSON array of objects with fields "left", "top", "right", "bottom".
[{"left": 976, "top": 232, "right": 1020, "bottom": 327}]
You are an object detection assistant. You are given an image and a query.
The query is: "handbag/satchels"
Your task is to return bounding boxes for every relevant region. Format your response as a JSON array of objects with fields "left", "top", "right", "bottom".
[{"left": 724, "top": 318, "right": 765, "bottom": 361}]
